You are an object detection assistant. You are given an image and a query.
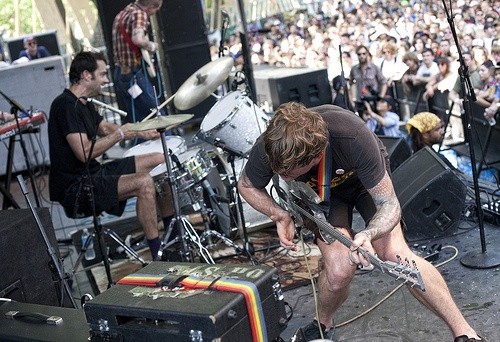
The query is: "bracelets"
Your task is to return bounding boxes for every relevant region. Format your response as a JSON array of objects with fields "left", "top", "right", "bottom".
[
  {"left": 118, "top": 128, "right": 124, "bottom": 141},
  {"left": 151, "top": 42, "right": 155, "bottom": 51}
]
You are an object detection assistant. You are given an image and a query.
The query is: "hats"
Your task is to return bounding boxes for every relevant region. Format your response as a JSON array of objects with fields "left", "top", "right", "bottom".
[
  {"left": 332, "top": 75, "right": 350, "bottom": 91},
  {"left": 484, "top": 23, "right": 498, "bottom": 32},
  {"left": 420, "top": 32, "right": 430, "bottom": 39},
  {"left": 462, "top": 6, "right": 472, "bottom": 12},
  {"left": 428, "top": 28, "right": 437, "bottom": 34},
  {"left": 485, "top": 13, "right": 496, "bottom": 21},
  {"left": 379, "top": 95, "right": 395, "bottom": 109},
  {"left": 471, "top": 38, "right": 485, "bottom": 48},
  {"left": 491, "top": 61, "right": 500, "bottom": 69}
]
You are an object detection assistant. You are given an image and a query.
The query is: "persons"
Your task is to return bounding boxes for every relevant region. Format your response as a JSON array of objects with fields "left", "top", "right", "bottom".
[
  {"left": 233, "top": 100, "right": 489, "bottom": 341},
  {"left": 110, "top": 0, "right": 171, "bottom": 140},
  {"left": 17, "top": 34, "right": 52, "bottom": 62},
  {"left": 1, "top": 107, "right": 26, "bottom": 126},
  {"left": 47, "top": 49, "right": 194, "bottom": 263},
  {"left": 203, "top": 0, "right": 500, "bottom": 168}
]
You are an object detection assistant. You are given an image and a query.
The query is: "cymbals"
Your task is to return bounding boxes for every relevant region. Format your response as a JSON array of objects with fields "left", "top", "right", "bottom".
[
  {"left": 173, "top": 56, "right": 234, "bottom": 110},
  {"left": 127, "top": 114, "right": 194, "bottom": 132}
]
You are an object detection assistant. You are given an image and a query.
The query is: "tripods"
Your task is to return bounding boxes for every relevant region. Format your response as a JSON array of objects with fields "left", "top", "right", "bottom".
[
  {"left": 129, "top": 113, "right": 215, "bottom": 267},
  {"left": 68, "top": 108, "right": 146, "bottom": 290}
]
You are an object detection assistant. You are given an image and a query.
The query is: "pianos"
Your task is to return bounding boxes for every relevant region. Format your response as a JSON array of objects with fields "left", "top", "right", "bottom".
[{"left": 0, "top": 106, "right": 46, "bottom": 211}]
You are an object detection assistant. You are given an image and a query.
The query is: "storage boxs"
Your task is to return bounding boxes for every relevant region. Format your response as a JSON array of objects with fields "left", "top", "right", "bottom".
[{"left": 84, "top": 260, "right": 287, "bottom": 342}]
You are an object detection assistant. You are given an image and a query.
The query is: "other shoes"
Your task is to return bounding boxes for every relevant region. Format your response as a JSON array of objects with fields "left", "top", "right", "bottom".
[
  {"left": 291, "top": 317, "right": 334, "bottom": 342},
  {"left": 454, "top": 333, "right": 487, "bottom": 342}
]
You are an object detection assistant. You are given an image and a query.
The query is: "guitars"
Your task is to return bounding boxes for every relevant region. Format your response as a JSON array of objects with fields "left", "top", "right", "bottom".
[
  {"left": 141, "top": 34, "right": 156, "bottom": 78},
  {"left": 278, "top": 177, "right": 426, "bottom": 292}
]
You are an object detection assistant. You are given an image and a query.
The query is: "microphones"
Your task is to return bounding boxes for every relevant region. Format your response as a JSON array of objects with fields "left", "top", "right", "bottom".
[{"left": 80, "top": 95, "right": 128, "bottom": 116}]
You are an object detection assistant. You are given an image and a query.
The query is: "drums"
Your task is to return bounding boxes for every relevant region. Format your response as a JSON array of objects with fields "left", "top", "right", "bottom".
[
  {"left": 148, "top": 146, "right": 213, "bottom": 198},
  {"left": 124, "top": 136, "right": 186, "bottom": 163},
  {"left": 200, "top": 91, "right": 271, "bottom": 158}
]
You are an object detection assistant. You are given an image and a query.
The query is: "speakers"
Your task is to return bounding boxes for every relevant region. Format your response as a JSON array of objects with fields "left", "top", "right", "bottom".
[
  {"left": 0, "top": 207, "right": 75, "bottom": 308},
  {"left": 253, "top": 66, "right": 333, "bottom": 114},
  {"left": 151, "top": 0, "right": 220, "bottom": 126},
  {"left": 378, "top": 135, "right": 412, "bottom": 175},
  {"left": 390, "top": 145, "right": 468, "bottom": 240}
]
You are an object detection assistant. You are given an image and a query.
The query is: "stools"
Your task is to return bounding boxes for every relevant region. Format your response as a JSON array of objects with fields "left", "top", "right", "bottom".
[{"left": 65, "top": 209, "right": 118, "bottom": 290}]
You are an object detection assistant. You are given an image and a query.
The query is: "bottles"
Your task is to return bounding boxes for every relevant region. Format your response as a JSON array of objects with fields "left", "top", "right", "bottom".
[
  {"left": 484, "top": 109, "right": 496, "bottom": 126},
  {"left": 80, "top": 229, "right": 95, "bottom": 260}
]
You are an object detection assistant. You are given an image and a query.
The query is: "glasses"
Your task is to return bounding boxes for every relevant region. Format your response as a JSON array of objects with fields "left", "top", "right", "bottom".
[
  {"left": 383, "top": 51, "right": 391, "bottom": 54},
  {"left": 358, "top": 52, "right": 367, "bottom": 56},
  {"left": 476, "top": 14, "right": 483, "bottom": 16}
]
